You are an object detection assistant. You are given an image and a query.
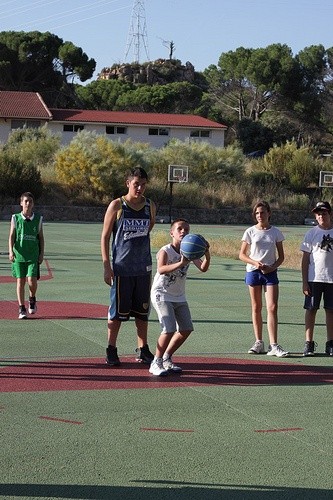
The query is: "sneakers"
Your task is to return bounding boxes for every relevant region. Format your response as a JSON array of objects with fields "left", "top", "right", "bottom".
[
  {"left": 105, "top": 345, "right": 120, "bottom": 366},
  {"left": 28, "top": 299, "right": 37, "bottom": 314},
  {"left": 148, "top": 358, "right": 168, "bottom": 376},
  {"left": 325, "top": 341, "right": 333, "bottom": 356},
  {"left": 247, "top": 341, "right": 264, "bottom": 354},
  {"left": 135, "top": 344, "right": 154, "bottom": 363},
  {"left": 163, "top": 357, "right": 182, "bottom": 373},
  {"left": 18, "top": 311, "right": 27, "bottom": 319},
  {"left": 303, "top": 341, "right": 318, "bottom": 356},
  {"left": 266, "top": 344, "right": 290, "bottom": 358}
]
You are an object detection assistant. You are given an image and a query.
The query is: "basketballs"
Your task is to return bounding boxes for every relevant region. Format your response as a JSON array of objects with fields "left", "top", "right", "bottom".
[{"left": 181, "top": 234, "right": 207, "bottom": 260}]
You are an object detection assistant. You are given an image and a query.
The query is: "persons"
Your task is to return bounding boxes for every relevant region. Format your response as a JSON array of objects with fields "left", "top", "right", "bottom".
[
  {"left": 300, "top": 201, "right": 333, "bottom": 356},
  {"left": 239, "top": 201, "right": 292, "bottom": 355},
  {"left": 101, "top": 167, "right": 156, "bottom": 367},
  {"left": 9, "top": 192, "right": 45, "bottom": 320},
  {"left": 149, "top": 218, "right": 210, "bottom": 375}
]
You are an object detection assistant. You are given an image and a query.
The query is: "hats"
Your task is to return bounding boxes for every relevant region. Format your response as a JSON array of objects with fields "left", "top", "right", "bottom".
[{"left": 311, "top": 201, "right": 332, "bottom": 212}]
